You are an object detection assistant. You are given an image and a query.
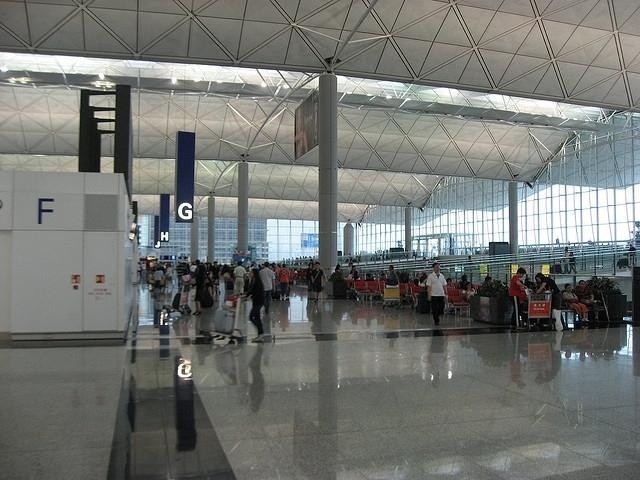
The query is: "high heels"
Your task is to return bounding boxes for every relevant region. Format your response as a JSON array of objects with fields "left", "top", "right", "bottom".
[
  {"left": 192, "top": 310, "right": 198, "bottom": 315},
  {"left": 196, "top": 311, "right": 202, "bottom": 316}
]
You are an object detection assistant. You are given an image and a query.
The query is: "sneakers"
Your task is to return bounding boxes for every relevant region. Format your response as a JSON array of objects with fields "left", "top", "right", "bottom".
[
  {"left": 199, "top": 330, "right": 212, "bottom": 336},
  {"left": 595, "top": 318, "right": 600, "bottom": 323},
  {"left": 582, "top": 318, "right": 589, "bottom": 322},
  {"left": 314, "top": 295, "right": 319, "bottom": 303},
  {"left": 516, "top": 326, "right": 526, "bottom": 331},
  {"left": 252, "top": 332, "right": 265, "bottom": 342},
  {"left": 281, "top": 295, "right": 288, "bottom": 300},
  {"left": 510, "top": 324, "right": 516, "bottom": 329}
]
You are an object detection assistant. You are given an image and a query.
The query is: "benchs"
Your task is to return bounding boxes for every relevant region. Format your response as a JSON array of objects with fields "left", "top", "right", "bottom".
[{"left": 521, "top": 298, "right": 609, "bottom": 331}]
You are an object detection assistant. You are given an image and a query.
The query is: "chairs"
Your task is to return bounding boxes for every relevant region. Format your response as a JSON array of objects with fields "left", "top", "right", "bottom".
[{"left": 345, "top": 278, "right": 483, "bottom": 319}]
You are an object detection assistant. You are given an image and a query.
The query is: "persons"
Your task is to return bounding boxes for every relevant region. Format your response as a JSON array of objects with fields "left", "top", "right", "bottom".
[
  {"left": 561, "top": 246, "right": 577, "bottom": 274},
  {"left": 294, "top": 105, "right": 309, "bottom": 159},
  {"left": 136, "top": 258, "right": 290, "bottom": 343},
  {"left": 511, "top": 331, "right": 616, "bottom": 389},
  {"left": 428, "top": 327, "right": 446, "bottom": 387},
  {"left": 627, "top": 244, "right": 638, "bottom": 265},
  {"left": 411, "top": 263, "right": 598, "bottom": 331},
  {"left": 244, "top": 345, "right": 265, "bottom": 412},
  {"left": 307, "top": 262, "right": 409, "bottom": 307}
]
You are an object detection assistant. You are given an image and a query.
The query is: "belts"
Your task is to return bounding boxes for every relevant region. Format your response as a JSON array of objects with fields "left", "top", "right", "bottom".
[{"left": 236, "top": 276, "right": 243, "bottom": 278}]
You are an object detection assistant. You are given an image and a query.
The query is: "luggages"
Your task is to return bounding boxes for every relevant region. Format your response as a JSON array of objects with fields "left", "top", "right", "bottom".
[
  {"left": 214, "top": 311, "right": 235, "bottom": 334},
  {"left": 417, "top": 290, "right": 431, "bottom": 315}
]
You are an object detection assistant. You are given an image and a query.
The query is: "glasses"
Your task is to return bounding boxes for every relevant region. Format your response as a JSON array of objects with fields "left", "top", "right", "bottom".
[
  {"left": 435, "top": 266, "right": 440, "bottom": 268},
  {"left": 468, "top": 285, "right": 471, "bottom": 286}
]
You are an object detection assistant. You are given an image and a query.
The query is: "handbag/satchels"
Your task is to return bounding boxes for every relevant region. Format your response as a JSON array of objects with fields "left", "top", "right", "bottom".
[
  {"left": 385, "top": 278, "right": 399, "bottom": 286},
  {"left": 567, "top": 298, "right": 577, "bottom": 304}
]
